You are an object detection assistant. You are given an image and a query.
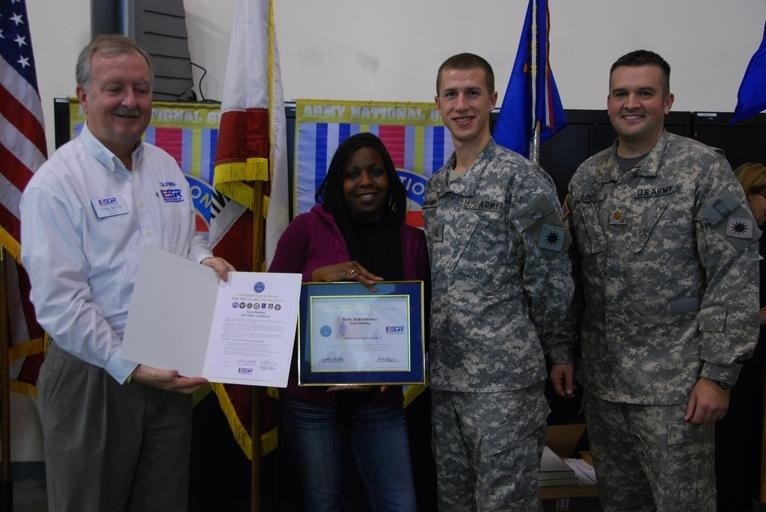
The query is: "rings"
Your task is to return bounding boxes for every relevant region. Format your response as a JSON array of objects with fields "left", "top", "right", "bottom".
[{"left": 349, "top": 268, "right": 358, "bottom": 275}]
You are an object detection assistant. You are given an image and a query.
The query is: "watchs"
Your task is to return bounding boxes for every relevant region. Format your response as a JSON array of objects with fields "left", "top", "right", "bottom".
[{"left": 711, "top": 379, "right": 733, "bottom": 391}]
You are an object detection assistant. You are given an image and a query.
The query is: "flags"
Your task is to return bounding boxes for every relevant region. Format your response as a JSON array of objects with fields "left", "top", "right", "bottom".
[
  {"left": 729, "top": 19, "right": 765, "bottom": 123},
  {"left": 202, "top": 0, "right": 302, "bottom": 462},
  {"left": 488, "top": 0, "right": 570, "bottom": 166},
  {"left": 0, "top": 0, "right": 53, "bottom": 407}
]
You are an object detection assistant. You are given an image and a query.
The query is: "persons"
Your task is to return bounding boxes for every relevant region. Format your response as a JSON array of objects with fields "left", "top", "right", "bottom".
[
  {"left": 717, "top": 157, "right": 764, "bottom": 512},
  {"left": 548, "top": 48, "right": 761, "bottom": 511},
  {"left": 266, "top": 132, "right": 435, "bottom": 512},
  {"left": 18, "top": 31, "right": 238, "bottom": 510},
  {"left": 418, "top": 52, "right": 577, "bottom": 511}
]
